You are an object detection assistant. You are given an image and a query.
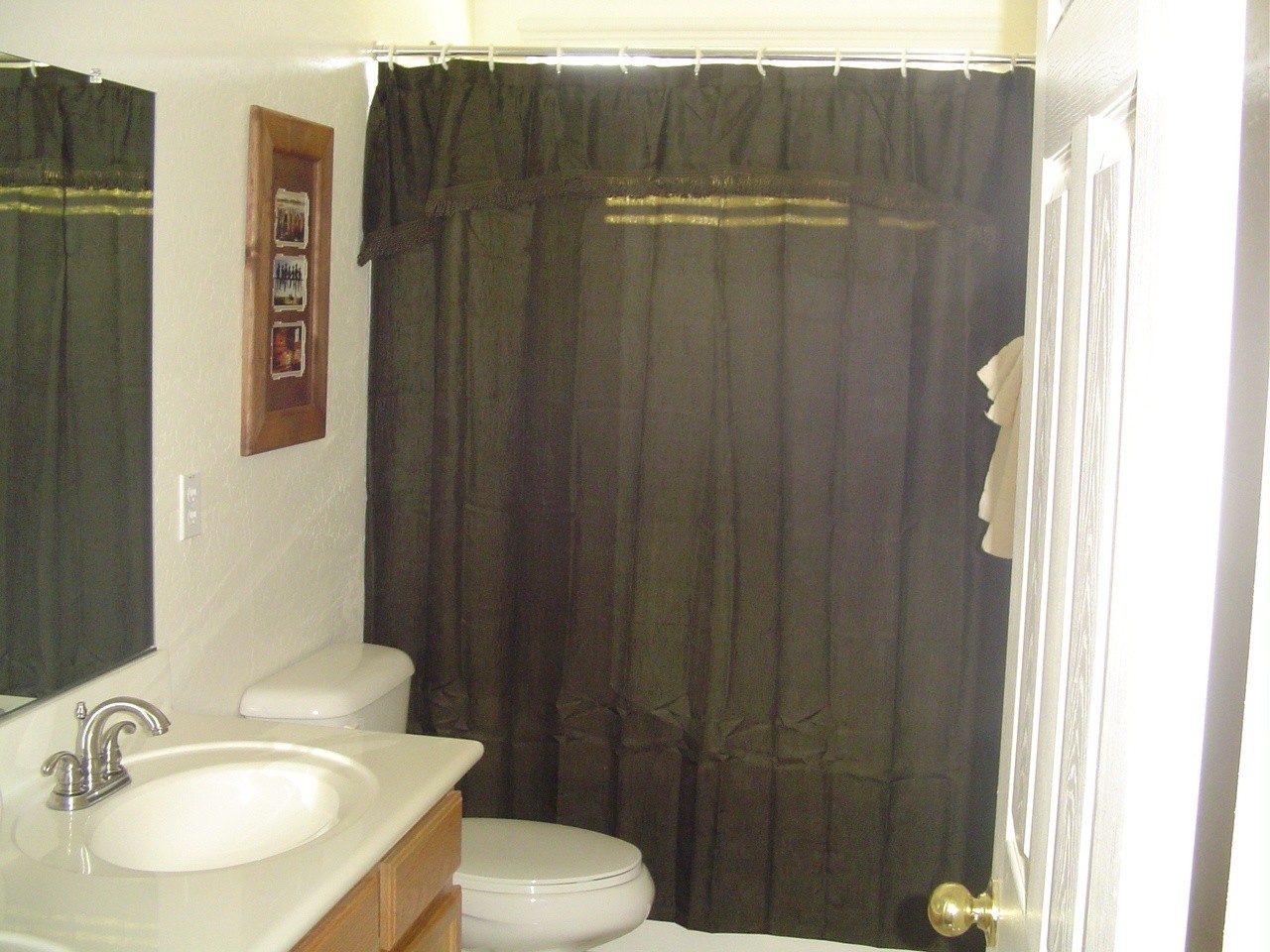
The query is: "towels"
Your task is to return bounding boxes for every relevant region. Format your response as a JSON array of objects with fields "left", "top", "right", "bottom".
[{"left": 975, "top": 334, "right": 1026, "bottom": 563}]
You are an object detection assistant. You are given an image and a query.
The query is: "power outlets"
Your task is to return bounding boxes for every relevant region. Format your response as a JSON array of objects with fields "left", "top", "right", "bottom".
[{"left": 179, "top": 471, "right": 202, "bottom": 540}]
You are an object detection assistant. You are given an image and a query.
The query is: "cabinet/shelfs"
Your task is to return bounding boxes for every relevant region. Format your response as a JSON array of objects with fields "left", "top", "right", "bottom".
[{"left": 287, "top": 786, "right": 463, "bottom": 952}]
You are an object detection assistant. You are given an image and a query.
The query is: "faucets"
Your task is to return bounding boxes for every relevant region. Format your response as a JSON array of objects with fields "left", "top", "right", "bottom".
[{"left": 40, "top": 695, "right": 170, "bottom": 812}]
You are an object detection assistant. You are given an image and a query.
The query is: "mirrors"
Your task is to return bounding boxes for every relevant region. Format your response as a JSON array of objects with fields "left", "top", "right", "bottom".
[{"left": 0, "top": 53, "right": 157, "bottom": 719}]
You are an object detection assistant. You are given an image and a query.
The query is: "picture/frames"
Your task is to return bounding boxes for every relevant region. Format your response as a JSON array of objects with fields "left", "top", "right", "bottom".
[{"left": 241, "top": 105, "right": 335, "bottom": 454}]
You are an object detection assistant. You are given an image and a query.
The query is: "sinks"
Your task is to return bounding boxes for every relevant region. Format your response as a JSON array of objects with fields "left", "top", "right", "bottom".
[{"left": 83, "top": 756, "right": 341, "bottom": 877}]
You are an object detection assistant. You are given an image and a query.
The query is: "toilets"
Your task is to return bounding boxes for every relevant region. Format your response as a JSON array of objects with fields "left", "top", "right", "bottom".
[{"left": 241, "top": 639, "right": 659, "bottom": 952}]
